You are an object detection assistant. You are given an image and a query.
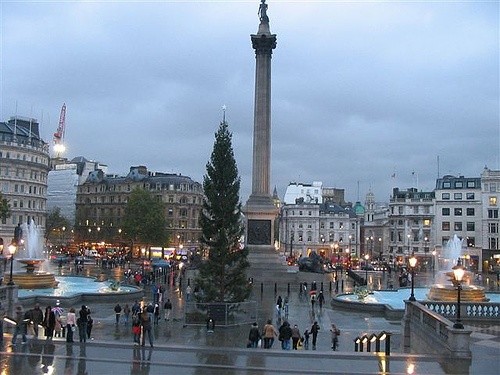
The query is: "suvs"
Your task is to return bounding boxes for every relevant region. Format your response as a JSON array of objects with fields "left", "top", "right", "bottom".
[{"left": 297, "top": 257, "right": 333, "bottom": 274}]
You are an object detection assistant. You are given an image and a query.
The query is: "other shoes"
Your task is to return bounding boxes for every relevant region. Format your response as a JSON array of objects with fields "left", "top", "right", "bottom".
[
  {"left": 141, "top": 344, "right": 146, "bottom": 346},
  {"left": 149, "top": 342, "right": 154, "bottom": 348}
]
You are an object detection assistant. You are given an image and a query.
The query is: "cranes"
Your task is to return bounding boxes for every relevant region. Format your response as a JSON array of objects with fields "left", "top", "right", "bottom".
[{"left": 52, "top": 102, "right": 67, "bottom": 144}]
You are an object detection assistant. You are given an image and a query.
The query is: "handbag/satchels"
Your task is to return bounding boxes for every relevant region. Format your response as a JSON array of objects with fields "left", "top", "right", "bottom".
[
  {"left": 278, "top": 334, "right": 283, "bottom": 341},
  {"left": 59, "top": 315, "right": 68, "bottom": 325},
  {"left": 132, "top": 326, "right": 140, "bottom": 334},
  {"left": 297, "top": 339, "right": 303, "bottom": 350},
  {"left": 72, "top": 326, "right": 77, "bottom": 332},
  {"left": 246, "top": 340, "right": 251, "bottom": 348},
  {"left": 258, "top": 339, "right": 262, "bottom": 348}
]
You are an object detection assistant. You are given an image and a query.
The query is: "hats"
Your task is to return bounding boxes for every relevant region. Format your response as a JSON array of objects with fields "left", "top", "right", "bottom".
[{"left": 253, "top": 322, "right": 257, "bottom": 327}]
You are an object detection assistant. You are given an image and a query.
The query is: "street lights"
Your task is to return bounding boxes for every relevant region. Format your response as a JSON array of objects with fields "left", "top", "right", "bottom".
[
  {"left": 408, "top": 253, "right": 417, "bottom": 301},
  {"left": 61, "top": 226, "right": 122, "bottom": 241},
  {"left": 348, "top": 234, "right": 353, "bottom": 262},
  {"left": 7, "top": 242, "right": 17, "bottom": 286},
  {"left": 451, "top": 257, "right": 467, "bottom": 330},
  {"left": 366, "top": 236, "right": 382, "bottom": 262}
]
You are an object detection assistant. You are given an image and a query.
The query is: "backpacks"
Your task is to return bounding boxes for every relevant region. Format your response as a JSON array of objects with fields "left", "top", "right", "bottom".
[{"left": 334, "top": 328, "right": 341, "bottom": 336}]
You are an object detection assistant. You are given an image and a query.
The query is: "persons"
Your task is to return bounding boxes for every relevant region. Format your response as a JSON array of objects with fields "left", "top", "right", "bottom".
[
  {"left": 30, "top": 252, "right": 187, "bottom": 346},
  {"left": 141, "top": 309, "right": 154, "bottom": 348},
  {"left": 211, "top": 312, "right": 216, "bottom": 331},
  {"left": 248, "top": 323, "right": 260, "bottom": 348},
  {"left": 261, "top": 291, "right": 340, "bottom": 350},
  {"left": 11, "top": 307, "right": 27, "bottom": 346},
  {"left": 207, "top": 311, "right": 211, "bottom": 332},
  {"left": 0, "top": 302, "right": 6, "bottom": 341}
]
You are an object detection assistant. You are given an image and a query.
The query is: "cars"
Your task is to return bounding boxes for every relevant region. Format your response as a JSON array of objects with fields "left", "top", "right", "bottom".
[{"left": 361, "top": 261, "right": 387, "bottom": 271}]
